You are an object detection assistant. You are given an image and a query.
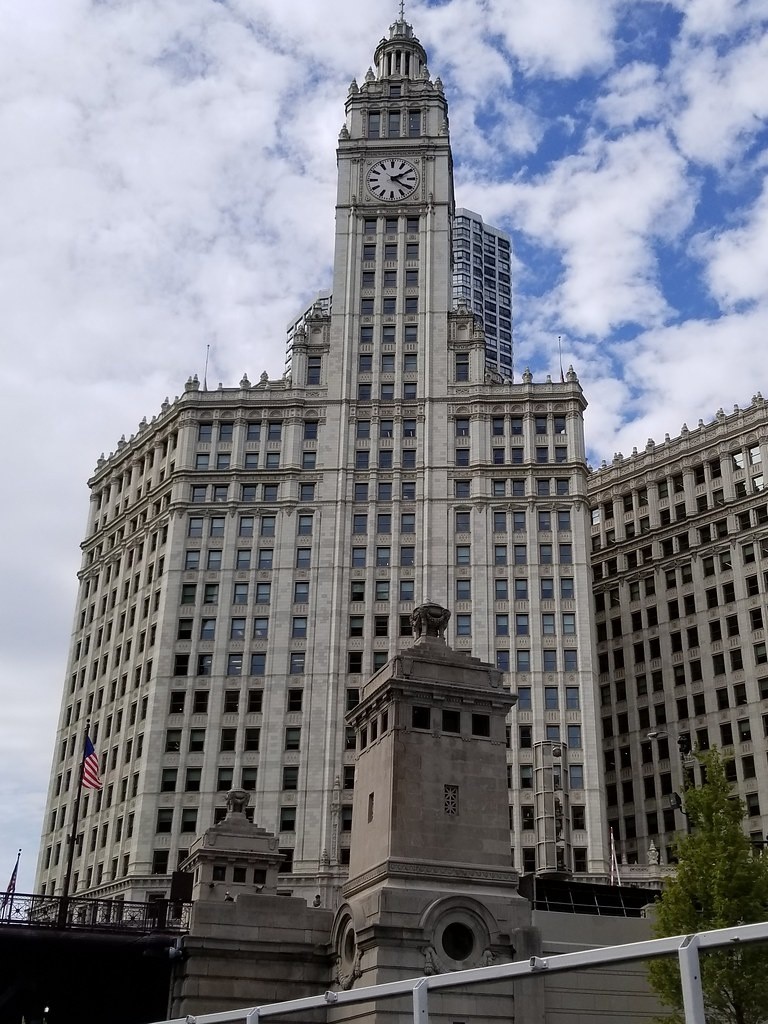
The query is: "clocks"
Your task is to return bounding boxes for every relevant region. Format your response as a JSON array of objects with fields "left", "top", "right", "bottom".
[{"left": 364, "top": 159, "right": 420, "bottom": 202}]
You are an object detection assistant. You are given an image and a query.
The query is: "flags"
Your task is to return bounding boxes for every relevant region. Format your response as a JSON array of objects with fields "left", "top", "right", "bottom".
[
  {"left": 0, "top": 861, "right": 17, "bottom": 910},
  {"left": 82, "top": 736, "right": 103, "bottom": 790}
]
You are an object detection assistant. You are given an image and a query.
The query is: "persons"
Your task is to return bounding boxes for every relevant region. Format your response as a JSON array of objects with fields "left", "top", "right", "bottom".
[
  {"left": 224, "top": 892, "right": 234, "bottom": 902},
  {"left": 313, "top": 895, "right": 320, "bottom": 908}
]
